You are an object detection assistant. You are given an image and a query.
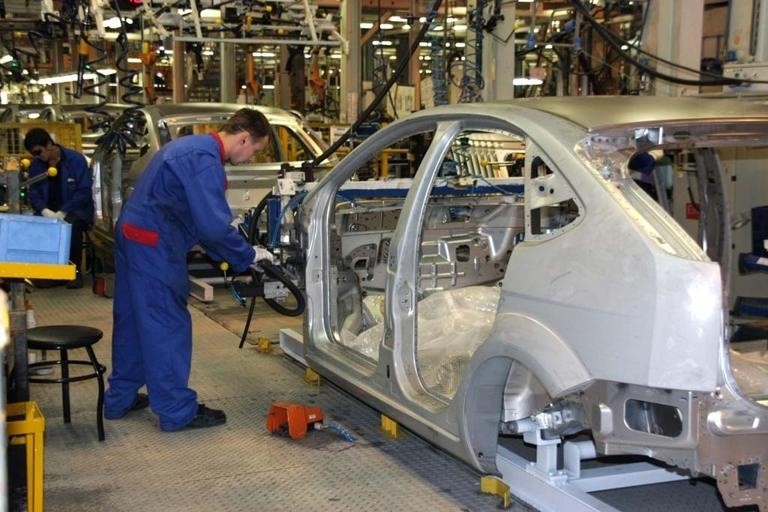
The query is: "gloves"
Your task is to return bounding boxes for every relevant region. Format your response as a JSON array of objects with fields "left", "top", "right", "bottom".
[
  {"left": 253, "top": 245, "right": 276, "bottom": 274},
  {"left": 41, "top": 209, "right": 67, "bottom": 219}
]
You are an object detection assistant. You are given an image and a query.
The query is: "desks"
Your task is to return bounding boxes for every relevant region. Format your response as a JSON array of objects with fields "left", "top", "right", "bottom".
[{"left": 1, "top": 262, "right": 81, "bottom": 402}]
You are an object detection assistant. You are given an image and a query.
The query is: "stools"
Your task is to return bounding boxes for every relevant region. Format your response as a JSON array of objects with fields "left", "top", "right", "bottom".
[{"left": 19, "top": 324, "right": 112, "bottom": 443}]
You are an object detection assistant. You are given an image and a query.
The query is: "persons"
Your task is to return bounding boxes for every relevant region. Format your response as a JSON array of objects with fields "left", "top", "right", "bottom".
[
  {"left": 103, "top": 108, "right": 273, "bottom": 432},
  {"left": 24, "top": 127, "right": 95, "bottom": 289}
]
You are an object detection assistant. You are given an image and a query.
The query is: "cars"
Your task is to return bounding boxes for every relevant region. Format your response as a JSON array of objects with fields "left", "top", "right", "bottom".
[
  {"left": 295, "top": 94, "right": 768, "bottom": 512},
  {"left": 86, "top": 101, "right": 344, "bottom": 274},
  {"left": 0, "top": 101, "right": 124, "bottom": 158}
]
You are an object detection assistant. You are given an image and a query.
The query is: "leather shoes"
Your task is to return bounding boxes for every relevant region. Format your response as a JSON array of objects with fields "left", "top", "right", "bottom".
[
  {"left": 163, "top": 403, "right": 225, "bottom": 430},
  {"left": 68, "top": 270, "right": 85, "bottom": 290},
  {"left": 103, "top": 394, "right": 148, "bottom": 419}
]
